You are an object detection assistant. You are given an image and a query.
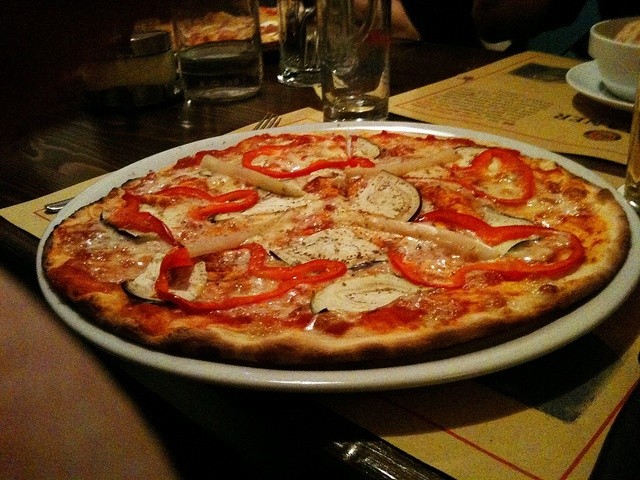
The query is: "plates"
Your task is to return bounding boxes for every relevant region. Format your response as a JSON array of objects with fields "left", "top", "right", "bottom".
[
  {"left": 565, "top": 61, "right": 634, "bottom": 110},
  {"left": 35, "top": 120, "right": 640, "bottom": 395}
]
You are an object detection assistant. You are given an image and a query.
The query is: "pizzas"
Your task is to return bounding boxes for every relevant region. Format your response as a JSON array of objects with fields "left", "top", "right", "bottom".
[
  {"left": 131, "top": 6, "right": 304, "bottom": 52},
  {"left": 41, "top": 128, "right": 631, "bottom": 364}
]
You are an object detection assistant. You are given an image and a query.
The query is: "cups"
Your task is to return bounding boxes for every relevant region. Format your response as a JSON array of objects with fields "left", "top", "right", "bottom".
[
  {"left": 276, "top": 0, "right": 320, "bottom": 88},
  {"left": 588, "top": 17, "right": 640, "bottom": 102},
  {"left": 322, "top": 0, "right": 391, "bottom": 122},
  {"left": 624, "top": 78, "right": 640, "bottom": 212},
  {"left": 174, "top": 0, "right": 264, "bottom": 105}
]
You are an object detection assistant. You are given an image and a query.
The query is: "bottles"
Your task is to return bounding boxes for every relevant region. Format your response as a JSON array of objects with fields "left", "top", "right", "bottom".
[{"left": 84, "top": 31, "right": 183, "bottom": 112}]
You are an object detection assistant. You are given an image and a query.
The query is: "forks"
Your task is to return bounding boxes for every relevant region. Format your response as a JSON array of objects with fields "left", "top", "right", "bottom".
[{"left": 45, "top": 112, "right": 282, "bottom": 212}]
